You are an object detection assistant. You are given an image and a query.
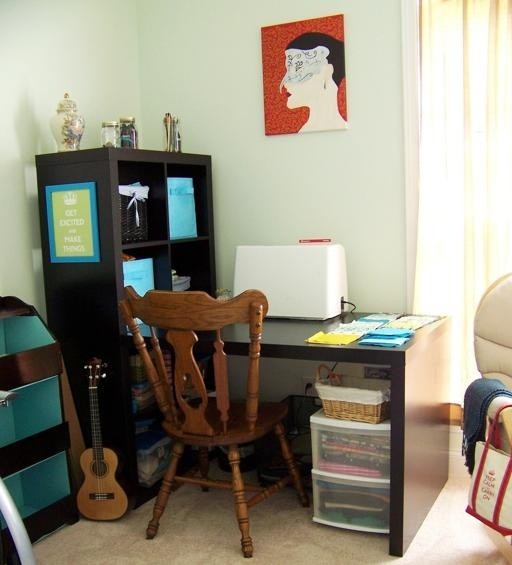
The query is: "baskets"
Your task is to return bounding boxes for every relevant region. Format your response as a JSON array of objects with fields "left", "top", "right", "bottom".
[
  {"left": 118, "top": 184, "right": 149, "bottom": 244},
  {"left": 314, "top": 364, "right": 390, "bottom": 425}
]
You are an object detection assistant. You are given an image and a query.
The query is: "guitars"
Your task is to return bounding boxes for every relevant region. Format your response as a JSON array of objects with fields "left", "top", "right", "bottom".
[{"left": 77, "top": 357, "right": 128, "bottom": 521}]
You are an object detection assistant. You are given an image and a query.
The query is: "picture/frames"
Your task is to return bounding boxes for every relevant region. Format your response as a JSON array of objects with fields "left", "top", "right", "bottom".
[
  {"left": 260, "top": 13, "right": 349, "bottom": 137},
  {"left": 44, "top": 181, "right": 102, "bottom": 264}
]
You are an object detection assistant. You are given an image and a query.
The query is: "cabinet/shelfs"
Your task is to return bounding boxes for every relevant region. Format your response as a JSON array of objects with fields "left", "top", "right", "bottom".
[
  {"left": 0, "top": 294, "right": 88, "bottom": 561},
  {"left": 34, "top": 146, "right": 217, "bottom": 509}
]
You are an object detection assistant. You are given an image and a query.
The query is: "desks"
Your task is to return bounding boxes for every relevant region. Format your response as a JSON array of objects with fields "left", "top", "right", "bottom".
[{"left": 122, "top": 310, "right": 452, "bottom": 558}]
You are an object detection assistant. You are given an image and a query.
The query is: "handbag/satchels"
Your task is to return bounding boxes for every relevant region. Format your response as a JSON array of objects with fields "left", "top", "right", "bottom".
[{"left": 464, "top": 405, "right": 512, "bottom": 537}]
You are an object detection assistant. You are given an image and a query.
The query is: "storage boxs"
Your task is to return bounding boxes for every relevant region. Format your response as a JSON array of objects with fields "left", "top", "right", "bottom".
[
  {"left": 309, "top": 407, "right": 391, "bottom": 481},
  {"left": 311, "top": 469, "right": 390, "bottom": 535}
]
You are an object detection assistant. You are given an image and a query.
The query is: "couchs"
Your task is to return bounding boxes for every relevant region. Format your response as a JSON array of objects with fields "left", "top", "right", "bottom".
[{"left": 472, "top": 271, "right": 512, "bottom": 565}]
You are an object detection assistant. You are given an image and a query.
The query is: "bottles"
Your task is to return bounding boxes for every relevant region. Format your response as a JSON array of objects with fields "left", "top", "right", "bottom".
[
  {"left": 101, "top": 121, "right": 120, "bottom": 148},
  {"left": 119, "top": 118, "right": 139, "bottom": 149}
]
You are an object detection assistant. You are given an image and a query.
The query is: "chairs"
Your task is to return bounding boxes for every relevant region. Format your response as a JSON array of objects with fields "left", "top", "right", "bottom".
[{"left": 117, "top": 284, "right": 311, "bottom": 558}]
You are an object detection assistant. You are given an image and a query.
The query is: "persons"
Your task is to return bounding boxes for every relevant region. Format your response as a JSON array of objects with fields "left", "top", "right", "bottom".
[{"left": 278, "top": 31, "right": 349, "bottom": 133}]
